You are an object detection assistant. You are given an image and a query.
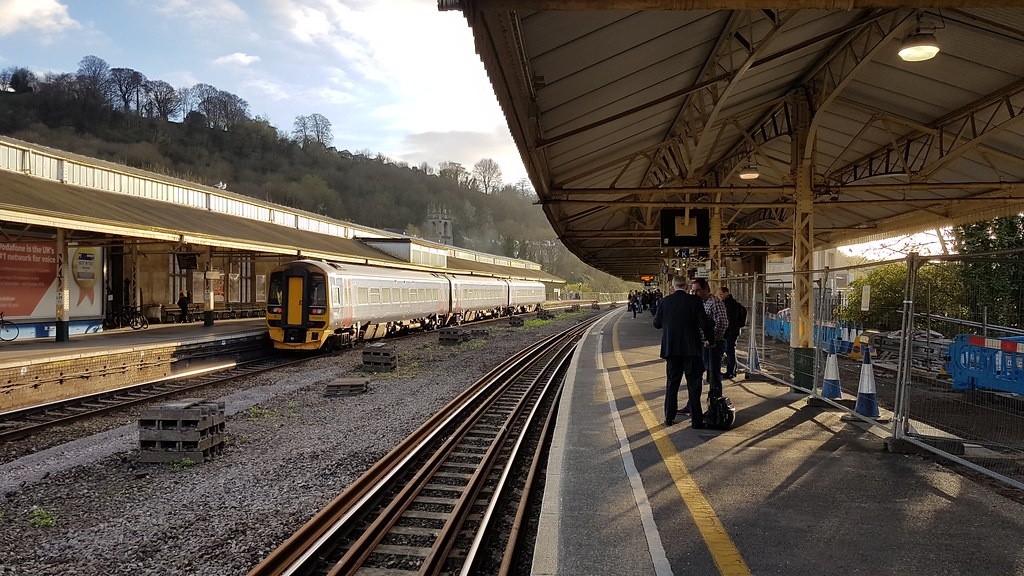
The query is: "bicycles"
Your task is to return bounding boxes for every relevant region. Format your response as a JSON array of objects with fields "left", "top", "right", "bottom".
[
  {"left": 0, "top": 312, "right": 20, "bottom": 342},
  {"left": 113, "top": 304, "right": 149, "bottom": 330}
]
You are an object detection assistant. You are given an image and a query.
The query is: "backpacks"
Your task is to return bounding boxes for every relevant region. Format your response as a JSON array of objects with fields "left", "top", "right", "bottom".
[
  {"left": 729, "top": 298, "right": 748, "bottom": 328},
  {"left": 703, "top": 396, "right": 735, "bottom": 430}
]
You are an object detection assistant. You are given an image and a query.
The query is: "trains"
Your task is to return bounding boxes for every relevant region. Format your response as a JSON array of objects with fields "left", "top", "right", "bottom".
[{"left": 263, "top": 250, "right": 547, "bottom": 350}]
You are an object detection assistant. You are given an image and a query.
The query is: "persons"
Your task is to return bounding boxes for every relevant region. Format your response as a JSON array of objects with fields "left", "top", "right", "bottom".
[
  {"left": 653, "top": 275, "right": 717, "bottom": 430},
  {"left": 717, "top": 286, "right": 747, "bottom": 379},
  {"left": 628, "top": 288, "right": 663, "bottom": 320},
  {"left": 669, "top": 278, "right": 729, "bottom": 423},
  {"left": 177, "top": 293, "right": 190, "bottom": 323}
]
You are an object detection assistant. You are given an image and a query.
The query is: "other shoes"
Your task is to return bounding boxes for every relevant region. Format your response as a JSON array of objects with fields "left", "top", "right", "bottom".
[
  {"left": 665, "top": 419, "right": 674, "bottom": 426},
  {"left": 691, "top": 422, "right": 707, "bottom": 429},
  {"left": 631, "top": 317, "right": 635, "bottom": 320},
  {"left": 721, "top": 373, "right": 734, "bottom": 379},
  {"left": 677, "top": 406, "right": 691, "bottom": 416},
  {"left": 703, "top": 410, "right": 709, "bottom": 419}
]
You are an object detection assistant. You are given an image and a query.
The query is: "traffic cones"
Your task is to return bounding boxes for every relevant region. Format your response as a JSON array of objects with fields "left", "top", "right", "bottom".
[
  {"left": 819, "top": 339, "right": 844, "bottom": 399},
  {"left": 840, "top": 346, "right": 892, "bottom": 422},
  {"left": 746, "top": 337, "right": 762, "bottom": 373}
]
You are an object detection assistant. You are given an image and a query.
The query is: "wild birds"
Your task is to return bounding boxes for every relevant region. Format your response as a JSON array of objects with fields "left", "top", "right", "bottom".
[{"left": 215, "top": 181, "right": 227, "bottom": 190}]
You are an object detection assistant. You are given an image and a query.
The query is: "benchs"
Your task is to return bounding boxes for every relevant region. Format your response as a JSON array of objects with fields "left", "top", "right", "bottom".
[{"left": 163, "top": 302, "right": 266, "bottom": 323}]
